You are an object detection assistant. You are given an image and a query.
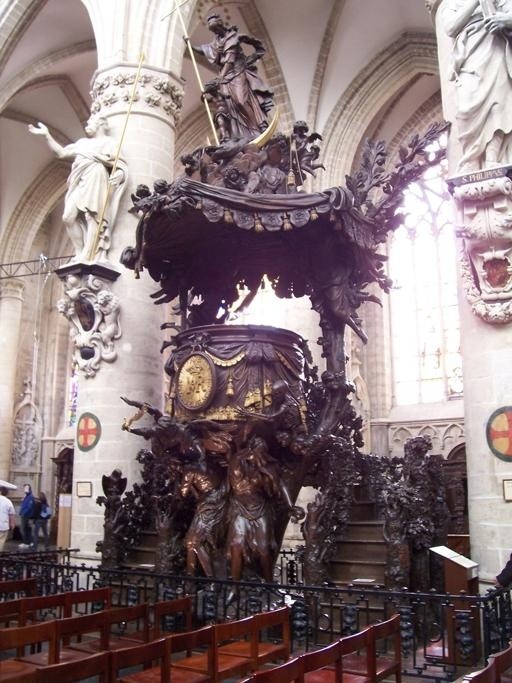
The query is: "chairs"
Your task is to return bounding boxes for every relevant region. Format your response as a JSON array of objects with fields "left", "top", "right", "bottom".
[{"left": 0, "top": 577, "right": 512, "bottom": 682}]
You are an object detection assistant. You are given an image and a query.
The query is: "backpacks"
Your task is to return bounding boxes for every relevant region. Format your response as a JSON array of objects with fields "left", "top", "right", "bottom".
[{"left": 40, "top": 506, "right": 52, "bottom": 519}]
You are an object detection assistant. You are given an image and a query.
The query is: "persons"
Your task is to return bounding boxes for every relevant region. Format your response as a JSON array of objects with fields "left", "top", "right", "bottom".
[
  {"left": 182, "top": 12, "right": 269, "bottom": 141},
  {"left": 494, "top": 552, "right": 512, "bottom": 588},
  {"left": 0, "top": 483, "right": 53, "bottom": 555},
  {"left": 174, "top": 379, "right": 305, "bottom": 595},
  {"left": 446, "top": 0, "right": 512, "bottom": 174},
  {"left": 28, "top": 113, "right": 126, "bottom": 262}
]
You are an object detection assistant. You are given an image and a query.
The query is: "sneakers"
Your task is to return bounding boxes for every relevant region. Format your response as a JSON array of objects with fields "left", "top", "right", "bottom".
[{"left": 18, "top": 542, "right": 50, "bottom": 548}]
